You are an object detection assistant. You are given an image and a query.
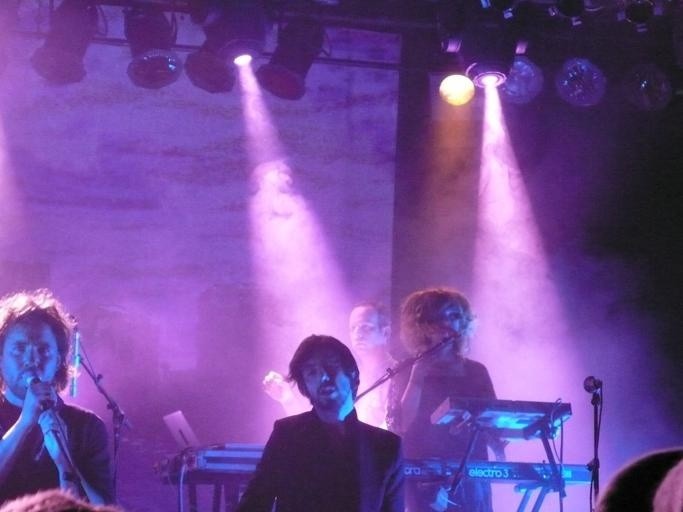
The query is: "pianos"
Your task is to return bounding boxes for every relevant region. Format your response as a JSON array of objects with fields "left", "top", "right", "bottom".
[{"left": 401, "top": 460, "right": 592, "bottom": 483}]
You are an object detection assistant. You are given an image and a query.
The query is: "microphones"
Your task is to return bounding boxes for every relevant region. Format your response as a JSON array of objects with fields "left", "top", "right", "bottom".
[
  {"left": 23, "top": 371, "right": 48, "bottom": 411},
  {"left": 410, "top": 337, "right": 453, "bottom": 364},
  {"left": 70, "top": 327, "right": 80, "bottom": 397},
  {"left": 584, "top": 376, "right": 602, "bottom": 393}
]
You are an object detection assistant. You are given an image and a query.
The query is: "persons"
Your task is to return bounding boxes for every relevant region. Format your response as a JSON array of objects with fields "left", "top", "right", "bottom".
[
  {"left": 2, "top": 289, "right": 112, "bottom": 508},
  {"left": 234, "top": 336, "right": 401, "bottom": 511},
  {"left": 386, "top": 287, "right": 510, "bottom": 512},
  {"left": 260, "top": 303, "right": 399, "bottom": 430}
]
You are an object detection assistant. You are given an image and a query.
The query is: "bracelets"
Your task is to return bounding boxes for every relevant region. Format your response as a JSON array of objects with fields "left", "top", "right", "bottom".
[{"left": 408, "top": 380, "right": 425, "bottom": 390}]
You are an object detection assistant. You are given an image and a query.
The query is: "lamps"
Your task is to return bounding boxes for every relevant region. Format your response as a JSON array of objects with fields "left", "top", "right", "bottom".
[
  {"left": 24, "top": 0, "right": 329, "bottom": 102},
  {"left": 415, "top": 0, "right": 668, "bottom": 108}
]
